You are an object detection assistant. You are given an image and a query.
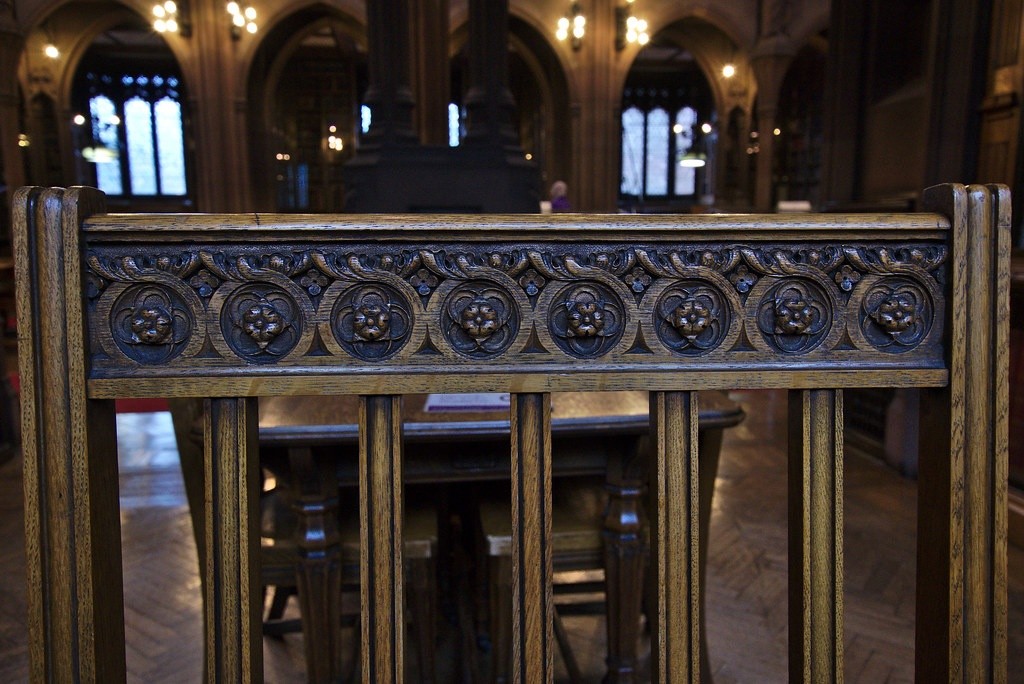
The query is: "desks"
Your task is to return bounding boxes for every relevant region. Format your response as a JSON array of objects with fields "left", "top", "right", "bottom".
[{"left": 189, "top": 390, "right": 748, "bottom": 684}]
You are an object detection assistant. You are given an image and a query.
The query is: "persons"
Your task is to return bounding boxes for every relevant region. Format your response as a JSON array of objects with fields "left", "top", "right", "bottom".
[{"left": 550, "top": 181, "right": 572, "bottom": 212}]
